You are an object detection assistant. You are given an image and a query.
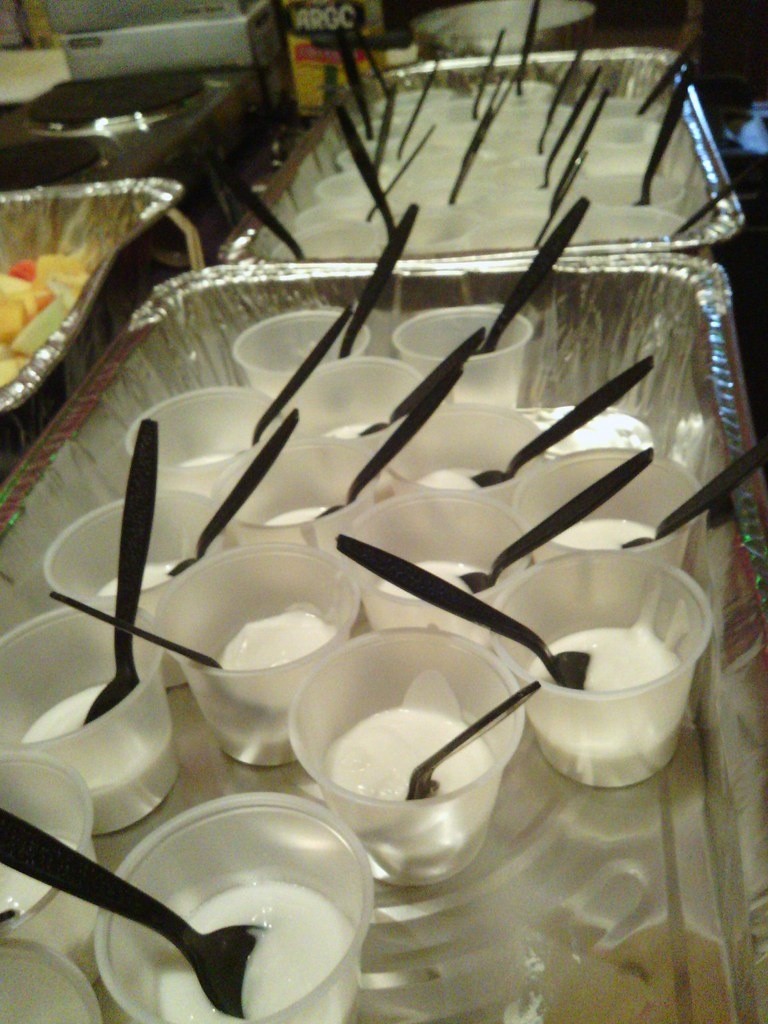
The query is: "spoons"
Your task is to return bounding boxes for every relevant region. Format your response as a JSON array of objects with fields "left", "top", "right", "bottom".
[
  {"left": 459, "top": 448, "right": 654, "bottom": 595},
  {"left": 335, "top": 535, "right": 590, "bottom": 690},
  {"left": 470, "top": 356, "right": 652, "bottom": 485},
  {"left": 0, "top": 807, "right": 266, "bottom": 1017},
  {"left": 82, "top": 419, "right": 159, "bottom": 729},
  {"left": 360, "top": 328, "right": 483, "bottom": 435},
  {"left": 625, "top": 445, "right": 768, "bottom": 550},
  {"left": 405, "top": 683, "right": 543, "bottom": 801},
  {"left": 321, "top": 369, "right": 466, "bottom": 522},
  {"left": 166, "top": 409, "right": 299, "bottom": 577}
]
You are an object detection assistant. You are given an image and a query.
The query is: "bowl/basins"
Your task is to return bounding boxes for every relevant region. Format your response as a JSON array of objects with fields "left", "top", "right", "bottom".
[{"left": 275, "top": 79, "right": 696, "bottom": 260}]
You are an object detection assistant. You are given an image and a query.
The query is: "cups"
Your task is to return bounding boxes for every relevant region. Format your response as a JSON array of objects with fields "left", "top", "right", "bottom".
[
  {"left": 0, "top": 604, "right": 178, "bottom": 834},
  {"left": 291, "top": 627, "right": 526, "bottom": 888},
  {"left": 517, "top": 447, "right": 701, "bottom": 572},
  {"left": 42, "top": 493, "right": 229, "bottom": 688},
  {"left": 387, "top": 403, "right": 539, "bottom": 489},
  {"left": 94, "top": 791, "right": 371, "bottom": 1024},
  {"left": 0, "top": 938, "right": 101, "bottom": 1024},
  {"left": 489, "top": 553, "right": 714, "bottom": 786},
  {"left": 285, "top": 355, "right": 426, "bottom": 436},
  {"left": 390, "top": 306, "right": 531, "bottom": 410},
  {"left": 0, "top": 752, "right": 104, "bottom": 1002},
  {"left": 124, "top": 388, "right": 279, "bottom": 491},
  {"left": 235, "top": 308, "right": 370, "bottom": 388},
  {"left": 347, "top": 493, "right": 527, "bottom": 630},
  {"left": 216, "top": 439, "right": 378, "bottom": 550},
  {"left": 156, "top": 544, "right": 359, "bottom": 766}
]
